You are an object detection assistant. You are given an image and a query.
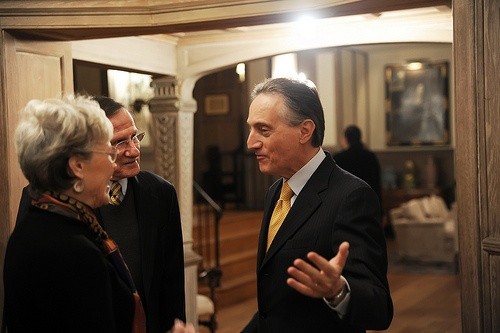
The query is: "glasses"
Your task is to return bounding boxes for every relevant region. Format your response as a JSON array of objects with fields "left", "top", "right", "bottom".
[
  {"left": 81, "top": 146, "right": 118, "bottom": 163},
  {"left": 110, "top": 127, "right": 146, "bottom": 150}
]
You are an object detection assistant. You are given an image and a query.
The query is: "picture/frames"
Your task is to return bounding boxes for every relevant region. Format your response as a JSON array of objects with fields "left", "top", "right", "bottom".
[
  {"left": 202, "top": 92, "right": 232, "bottom": 117},
  {"left": 384, "top": 60, "right": 452, "bottom": 150}
]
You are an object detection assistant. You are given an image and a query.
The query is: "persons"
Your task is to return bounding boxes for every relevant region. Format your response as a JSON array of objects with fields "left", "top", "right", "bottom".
[
  {"left": 239, "top": 78, "right": 395, "bottom": 333},
  {"left": 91, "top": 95, "right": 196, "bottom": 333},
  {"left": 0, "top": 93, "right": 146, "bottom": 333},
  {"left": 332, "top": 120, "right": 386, "bottom": 216}
]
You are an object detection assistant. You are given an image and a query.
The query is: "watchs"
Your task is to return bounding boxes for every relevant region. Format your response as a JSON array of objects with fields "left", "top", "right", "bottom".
[{"left": 320, "top": 283, "right": 350, "bottom": 311}]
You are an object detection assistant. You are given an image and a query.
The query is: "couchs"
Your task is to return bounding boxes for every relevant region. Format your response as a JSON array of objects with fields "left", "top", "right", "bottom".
[{"left": 390, "top": 195, "right": 457, "bottom": 265}]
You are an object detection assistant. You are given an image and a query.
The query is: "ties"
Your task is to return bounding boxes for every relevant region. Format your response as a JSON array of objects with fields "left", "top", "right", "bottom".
[
  {"left": 266, "top": 181, "right": 295, "bottom": 256},
  {"left": 109, "top": 181, "right": 122, "bottom": 207}
]
